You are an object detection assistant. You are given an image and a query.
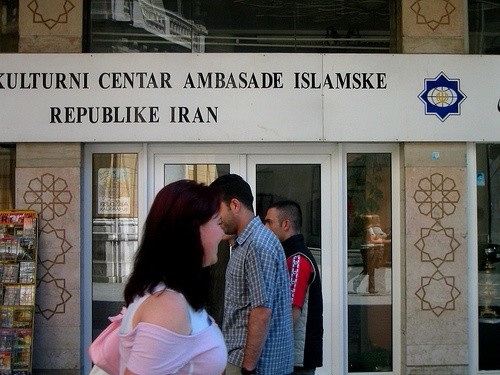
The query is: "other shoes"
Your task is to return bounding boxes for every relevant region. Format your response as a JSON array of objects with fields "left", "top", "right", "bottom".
[
  {"left": 368, "top": 289, "right": 378, "bottom": 293},
  {"left": 352, "top": 280, "right": 358, "bottom": 293}
]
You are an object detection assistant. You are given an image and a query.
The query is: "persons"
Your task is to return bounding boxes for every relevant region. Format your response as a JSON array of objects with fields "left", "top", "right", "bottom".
[
  {"left": 208, "top": 173, "right": 297, "bottom": 375},
  {"left": 346, "top": 215, "right": 393, "bottom": 296},
  {"left": 263, "top": 198, "right": 326, "bottom": 375},
  {"left": 114, "top": 179, "right": 231, "bottom": 375}
]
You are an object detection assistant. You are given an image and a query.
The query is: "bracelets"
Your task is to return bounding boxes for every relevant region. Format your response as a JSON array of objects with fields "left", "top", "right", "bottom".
[{"left": 240, "top": 367, "right": 256, "bottom": 375}]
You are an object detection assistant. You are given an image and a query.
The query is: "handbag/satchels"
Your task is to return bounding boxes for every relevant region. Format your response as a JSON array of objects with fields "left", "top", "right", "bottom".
[{"left": 90, "top": 306, "right": 127, "bottom": 374}]
